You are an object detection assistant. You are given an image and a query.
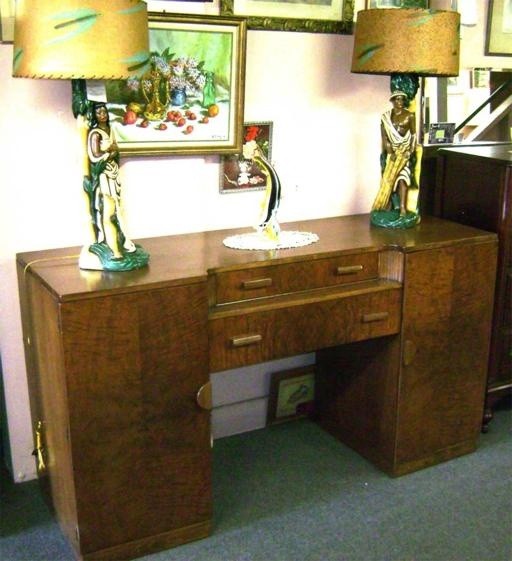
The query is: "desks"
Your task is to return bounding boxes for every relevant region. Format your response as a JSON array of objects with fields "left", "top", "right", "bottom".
[{"left": 14, "top": 212, "right": 500, "bottom": 561}]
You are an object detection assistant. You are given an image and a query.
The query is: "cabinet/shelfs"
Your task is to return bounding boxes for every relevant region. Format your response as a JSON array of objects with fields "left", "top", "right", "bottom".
[{"left": 432, "top": 147, "right": 512, "bottom": 434}]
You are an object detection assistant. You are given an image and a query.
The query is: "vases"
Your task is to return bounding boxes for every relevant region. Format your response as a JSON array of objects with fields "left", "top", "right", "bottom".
[
  {"left": 140, "top": 77, "right": 170, "bottom": 121},
  {"left": 168, "top": 86, "right": 186, "bottom": 105},
  {"left": 203, "top": 77, "right": 215, "bottom": 108}
]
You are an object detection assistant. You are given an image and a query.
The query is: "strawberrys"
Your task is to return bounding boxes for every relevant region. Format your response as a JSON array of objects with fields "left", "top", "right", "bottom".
[{"left": 141, "top": 109, "right": 209, "bottom": 132}]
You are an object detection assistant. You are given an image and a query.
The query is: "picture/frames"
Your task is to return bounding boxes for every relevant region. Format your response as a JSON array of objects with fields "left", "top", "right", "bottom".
[
  {"left": 484, "top": 0, "right": 512, "bottom": 56},
  {"left": 218, "top": 121, "right": 274, "bottom": 195},
  {"left": 266, "top": 364, "right": 317, "bottom": 427},
  {"left": 104, "top": 13, "right": 248, "bottom": 158},
  {"left": 219, "top": 0, "right": 356, "bottom": 35},
  {"left": 365, "top": 0, "right": 430, "bottom": 11}
]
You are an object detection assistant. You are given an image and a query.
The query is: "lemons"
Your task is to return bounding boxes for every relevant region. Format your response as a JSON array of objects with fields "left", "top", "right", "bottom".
[
  {"left": 208, "top": 105, "right": 218, "bottom": 117},
  {"left": 127, "top": 102, "right": 143, "bottom": 113}
]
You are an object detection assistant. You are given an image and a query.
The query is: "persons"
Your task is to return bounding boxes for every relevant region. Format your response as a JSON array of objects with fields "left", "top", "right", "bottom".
[
  {"left": 380, "top": 90, "right": 417, "bottom": 217},
  {"left": 89, "top": 105, "right": 133, "bottom": 260}
]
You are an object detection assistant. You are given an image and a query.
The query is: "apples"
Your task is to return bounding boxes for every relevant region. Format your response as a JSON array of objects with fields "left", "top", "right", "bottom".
[{"left": 124, "top": 112, "right": 136, "bottom": 125}]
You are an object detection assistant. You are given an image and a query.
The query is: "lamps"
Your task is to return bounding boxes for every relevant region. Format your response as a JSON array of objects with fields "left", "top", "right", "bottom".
[
  {"left": 350, "top": 7, "right": 460, "bottom": 230},
  {"left": 12, "top": 0, "right": 151, "bottom": 272}
]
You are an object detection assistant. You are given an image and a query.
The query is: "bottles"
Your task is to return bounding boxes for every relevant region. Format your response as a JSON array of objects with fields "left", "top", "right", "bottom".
[{"left": 201, "top": 71, "right": 216, "bottom": 108}]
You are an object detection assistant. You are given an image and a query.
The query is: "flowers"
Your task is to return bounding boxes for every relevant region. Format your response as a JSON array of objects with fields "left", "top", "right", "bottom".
[
  {"left": 126, "top": 46, "right": 206, "bottom": 98},
  {"left": 243, "top": 126, "right": 260, "bottom": 142}
]
